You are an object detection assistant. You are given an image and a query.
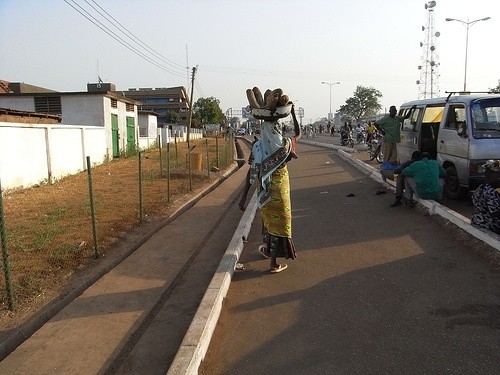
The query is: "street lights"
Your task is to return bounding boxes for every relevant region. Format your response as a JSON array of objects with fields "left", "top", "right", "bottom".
[
  {"left": 321, "top": 81, "right": 340, "bottom": 118},
  {"left": 445, "top": 16, "right": 491, "bottom": 92}
]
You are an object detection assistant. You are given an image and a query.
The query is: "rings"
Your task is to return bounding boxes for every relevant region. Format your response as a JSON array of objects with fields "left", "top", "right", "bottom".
[{"left": 240, "top": 207, "right": 241, "bottom": 208}]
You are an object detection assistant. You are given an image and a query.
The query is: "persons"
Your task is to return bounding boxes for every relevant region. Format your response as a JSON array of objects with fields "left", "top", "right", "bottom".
[
  {"left": 239, "top": 102, "right": 302, "bottom": 273},
  {"left": 283, "top": 105, "right": 500, "bottom": 235}
]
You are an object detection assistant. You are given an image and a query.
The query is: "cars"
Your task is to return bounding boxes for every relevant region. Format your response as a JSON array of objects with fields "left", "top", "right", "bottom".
[{"left": 236, "top": 126, "right": 260, "bottom": 136}]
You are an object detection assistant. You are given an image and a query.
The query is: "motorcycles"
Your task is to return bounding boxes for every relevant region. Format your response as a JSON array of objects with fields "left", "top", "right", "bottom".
[
  {"left": 335, "top": 120, "right": 385, "bottom": 149},
  {"left": 367, "top": 132, "right": 385, "bottom": 163}
]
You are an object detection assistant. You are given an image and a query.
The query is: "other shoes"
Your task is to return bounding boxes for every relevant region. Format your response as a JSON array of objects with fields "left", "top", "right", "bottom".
[
  {"left": 405, "top": 199, "right": 415, "bottom": 209},
  {"left": 384, "top": 198, "right": 403, "bottom": 208},
  {"left": 375, "top": 190, "right": 386, "bottom": 195}
]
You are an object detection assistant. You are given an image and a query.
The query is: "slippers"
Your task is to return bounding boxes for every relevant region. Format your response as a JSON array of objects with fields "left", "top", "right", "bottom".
[
  {"left": 258, "top": 244, "right": 272, "bottom": 259},
  {"left": 270, "top": 263, "right": 288, "bottom": 273}
]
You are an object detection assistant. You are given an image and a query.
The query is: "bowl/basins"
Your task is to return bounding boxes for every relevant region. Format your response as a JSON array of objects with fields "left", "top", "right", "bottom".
[{"left": 251, "top": 103, "right": 292, "bottom": 120}]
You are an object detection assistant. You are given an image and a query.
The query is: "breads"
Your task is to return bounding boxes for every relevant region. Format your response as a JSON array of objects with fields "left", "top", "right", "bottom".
[{"left": 246, "top": 87, "right": 289, "bottom": 108}]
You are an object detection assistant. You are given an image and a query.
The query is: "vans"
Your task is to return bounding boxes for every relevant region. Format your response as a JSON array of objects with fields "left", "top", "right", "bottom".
[{"left": 395, "top": 92, "right": 500, "bottom": 201}]
